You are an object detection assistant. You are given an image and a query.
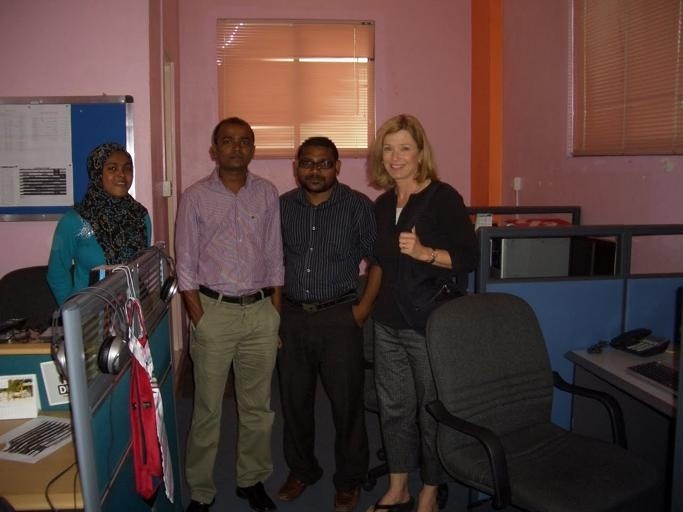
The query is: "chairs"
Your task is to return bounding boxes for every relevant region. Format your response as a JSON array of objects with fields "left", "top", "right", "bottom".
[
  {"left": 424, "top": 291, "right": 660, "bottom": 512},
  {"left": 0, "top": 265, "right": 63, "bottom": 333}
]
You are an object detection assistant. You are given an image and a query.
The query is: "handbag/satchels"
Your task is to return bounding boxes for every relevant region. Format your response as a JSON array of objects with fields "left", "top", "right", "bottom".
[{"left": 388, "top": 182, "right": 468, "bottom": 336}]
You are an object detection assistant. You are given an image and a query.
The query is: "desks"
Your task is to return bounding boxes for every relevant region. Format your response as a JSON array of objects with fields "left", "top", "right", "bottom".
[
  {"left": 567, "top": 339, "right": 683, "bottom": 512},
  {"left": 0, "top": 315, "right": 100, "bottom": 512}
]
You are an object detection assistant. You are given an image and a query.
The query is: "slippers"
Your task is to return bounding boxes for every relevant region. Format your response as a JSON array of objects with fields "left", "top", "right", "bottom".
[{"left": 367, "top": 492, "right": 416, "bottom": 512}]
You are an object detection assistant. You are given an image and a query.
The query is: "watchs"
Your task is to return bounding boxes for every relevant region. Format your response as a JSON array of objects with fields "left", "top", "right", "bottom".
[{"left": 428, "top": 246, "right": 439, "bottom": 267}]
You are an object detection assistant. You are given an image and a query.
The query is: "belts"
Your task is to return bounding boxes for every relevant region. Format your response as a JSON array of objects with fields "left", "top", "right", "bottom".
[
  {"left": 196, "top": 284, "right": 276, "bottom": 307},
  {"left": 279, "top": 290, "right": 360, "bottom": 314}
]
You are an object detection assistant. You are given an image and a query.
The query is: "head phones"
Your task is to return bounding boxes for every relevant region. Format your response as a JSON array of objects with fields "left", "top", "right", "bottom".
[
  {"left": 133, "top": 246, "right": 179, "bottom": 303},
  {"left": 52, "top": 285, "right": 129, "bottom": 377}
]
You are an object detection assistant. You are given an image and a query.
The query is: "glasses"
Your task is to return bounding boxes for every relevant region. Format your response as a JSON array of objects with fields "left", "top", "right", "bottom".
[{"left": 296, "top": 157, "right": 337, "bottom": 172}]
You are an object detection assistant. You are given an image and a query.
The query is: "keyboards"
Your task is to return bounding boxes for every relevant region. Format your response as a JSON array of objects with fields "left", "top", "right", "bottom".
[{"left": 628, "top": 360, "right": 678, "bottom": 395}]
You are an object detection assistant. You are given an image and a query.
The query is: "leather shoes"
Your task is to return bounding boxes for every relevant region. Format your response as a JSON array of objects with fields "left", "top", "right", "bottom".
[
  {"left": 333, "top": 481, "right": 360, "bottom": 511},
  {"left": 273, "top": 469, "right": 306, "bottom": 502},
  {"left": 181, "top": 494, "right": 218, "bottom": 512},
  {"left": 234, "top": 480, "right": 278, "bottom": 512}
]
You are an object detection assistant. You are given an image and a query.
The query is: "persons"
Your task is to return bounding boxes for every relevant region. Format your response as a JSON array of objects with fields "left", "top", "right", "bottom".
[
  {"left": 279, "top": 137, "right": 383, "bottom": 511},
  {"left": 367, "top": 116, "right": 482, "bottom": 511},
  {"left": 173, "top": 117, "right": 285, "bottom": 511},
  {"left": 45, "top": 143, "right": 151, "bottom": 306}
]
